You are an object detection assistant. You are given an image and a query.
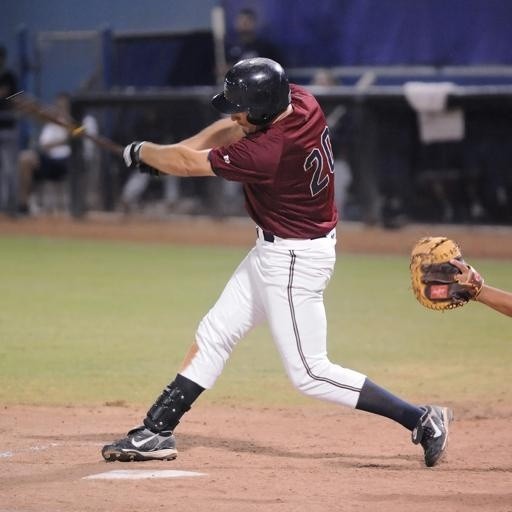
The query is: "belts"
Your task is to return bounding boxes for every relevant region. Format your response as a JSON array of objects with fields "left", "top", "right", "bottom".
[{"left": 255, "top": 226, "right": 326, "bottom": 243}]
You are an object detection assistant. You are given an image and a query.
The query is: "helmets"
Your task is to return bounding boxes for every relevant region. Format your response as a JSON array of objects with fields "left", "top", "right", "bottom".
[{"left": 212, "top": 58, "right": 288, "bottom": 122}]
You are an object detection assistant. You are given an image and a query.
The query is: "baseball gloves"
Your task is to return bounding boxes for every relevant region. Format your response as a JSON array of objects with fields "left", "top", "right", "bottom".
[{"left": 408, "top": 238, "right": 482, "bottom": 310}]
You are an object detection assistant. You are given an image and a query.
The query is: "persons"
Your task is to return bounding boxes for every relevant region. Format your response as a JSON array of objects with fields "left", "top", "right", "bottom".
[
  {"left": 226, "top": 9, "right": 277, "bottom": 64},
  {"left": 354, "top": 166, "right": 509, "bottom": 228},
  {"left": 122, "top": 101, "right": 182, "bottom": 215},
  {"left": 1, "top": 46, "right": 22, "bottom": 213},
  {"left": 102, "top": 56, "right": 452, "bottom": 466},
  {"left": 447, "top": 248, "right": 511, "bottom": 319},
  {"left": 311, "top": 69, "right": 366, "bottom": 222},
  {"left": 23, "top": 92, "right": 98, "bottom": 217}
]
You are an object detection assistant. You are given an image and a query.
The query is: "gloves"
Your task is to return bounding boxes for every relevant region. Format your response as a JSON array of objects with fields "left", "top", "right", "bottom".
[{"left": 122, "top": 140, "right": 147, "bottom": 169}]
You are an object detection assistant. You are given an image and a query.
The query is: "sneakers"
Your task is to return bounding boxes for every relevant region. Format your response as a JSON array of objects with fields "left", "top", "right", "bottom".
[
  {"left": 101, "top": 424, "right": 177, "bottom": 462},
  {"left": 412, "top": 405, "right": 455, "bottom": 467}
]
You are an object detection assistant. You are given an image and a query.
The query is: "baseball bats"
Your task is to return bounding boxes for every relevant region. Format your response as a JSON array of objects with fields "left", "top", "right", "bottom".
[{"left": 0, "top": 90, "right": 123, "bottom": 157}]
